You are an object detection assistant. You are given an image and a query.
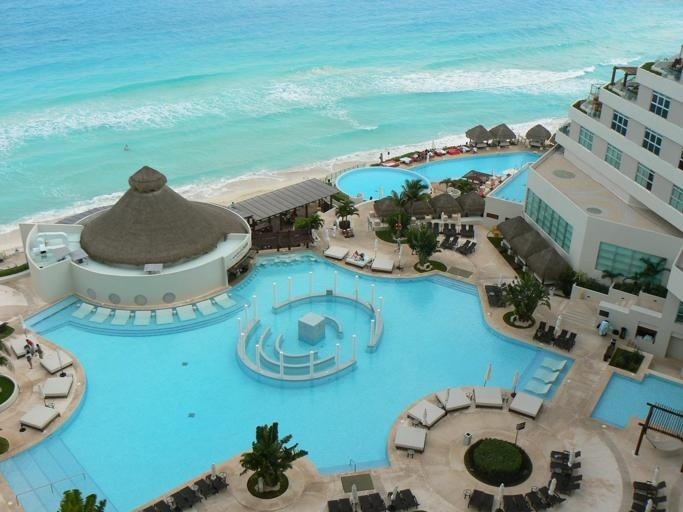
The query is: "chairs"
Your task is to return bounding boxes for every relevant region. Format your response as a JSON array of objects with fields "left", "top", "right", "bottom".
[
  {"left": 531, "top": 320, "right": 577, "bottom": 352},
  {"left": 427, "top": 222, "right": 477, "bottom": 257},
  {"left": 143, "top": 472, "right": 230, "bottom": 511},
  {"left": 524, "top": 356, "right": 568, "bottom": 395},
  {"left": 465, "top": 449, "right": 582, "bottom": 511},
  {"left": 69, "top": 293, "right": 237, "bottom": 327},
  {"left": 389, "top": 487, "right": 420, "bottom": 512},
  {"left": 629, "top": 479, "right": 668, "bottom": 512}
]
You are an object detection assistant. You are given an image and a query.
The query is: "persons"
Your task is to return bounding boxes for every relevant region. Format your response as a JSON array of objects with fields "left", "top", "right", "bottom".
[
  {"left": 22, "top": 338, "right": 44, "bottom": 370},
  {"left": 329, "top": 223, "right": 338, "bottom": 238},
  {"left": 350, "top": 250, "right": 366, "bottom": 262}
]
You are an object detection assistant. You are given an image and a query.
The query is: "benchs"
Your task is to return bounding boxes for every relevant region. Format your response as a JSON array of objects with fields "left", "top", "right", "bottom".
[
  {"left": 326, "top": 491, "right": 387, "bottom": 512},
  {"left": 322, "top": 246, "right": 394, "bottom": 274},
  {"left": 10, "top": 336, "right": 73, "bottom": 433},
  {"left": 393, "top": 388, "right": 543, "bottom": 452}
]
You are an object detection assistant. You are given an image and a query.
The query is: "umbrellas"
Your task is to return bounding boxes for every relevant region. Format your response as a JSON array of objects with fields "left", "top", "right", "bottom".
[
  {"left": 491, "top": 482, "right": 505, "bottom": 512},
  {"left": 55, "top": 346, "right": 64, "bottom": 375},
  {"left": 421, "top": 408, "right": 427, "bottom": 427},
  {"left": 441, "top": 387, "right": 454, "bottom": 409},
  {"left": 510, "top": 371, "right": 520, "bottom": 395},
  {"left": 553, "top": 317, "right": 562, "bottom": 338},
  {"left": 652, "top": 466, "right": 661, "bottom": 487},
  {"left": 372, "top": 237, "right": 378, "bottom": 261},
  {"left": 644, "top": 498, "right": 653, "bottom": 512},
  {"left": 349, "top": 480, "right": 360, "bottom": 512},
  {"left": 546, "top": 477, "right": 559, "bottom": 502},
  {"left": 395, "top": 240, "right": 401, "bottom": 268},
  {"left": 36, "top": 383, "right": 48, "bottom": 407},
  {"left": 483, "top": 362, "right": 493, "bottom": 382}
]
